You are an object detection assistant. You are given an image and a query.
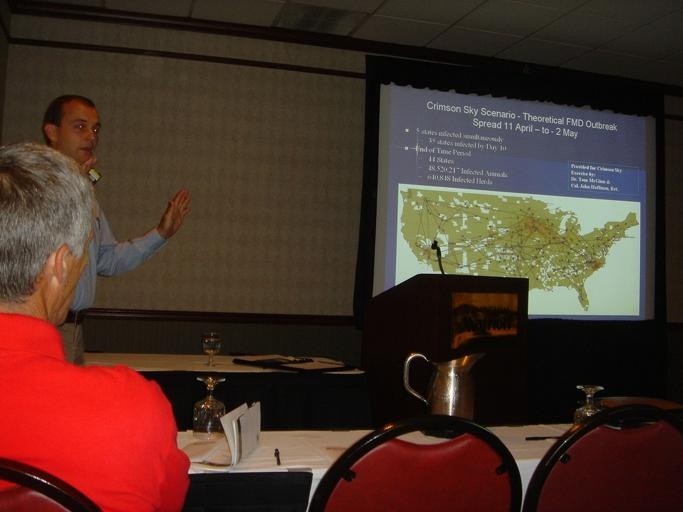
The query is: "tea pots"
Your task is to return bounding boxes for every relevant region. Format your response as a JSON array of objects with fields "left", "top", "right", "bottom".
[{"left": 402, "top": 351, "right": 482, "bottom": 420}]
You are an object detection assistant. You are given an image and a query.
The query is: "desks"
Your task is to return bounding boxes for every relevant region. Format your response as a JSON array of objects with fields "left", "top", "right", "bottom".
[
  {"left": 83, "top": 352, "right": 367, "bottom": 431},
  {"left": 176, "top": 424, "right": 620, "bottom": 509}
]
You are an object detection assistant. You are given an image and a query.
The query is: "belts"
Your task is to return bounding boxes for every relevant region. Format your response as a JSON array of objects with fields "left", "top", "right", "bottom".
[{"left": 62, "top": 309, "right": 81, "bottom": 327}]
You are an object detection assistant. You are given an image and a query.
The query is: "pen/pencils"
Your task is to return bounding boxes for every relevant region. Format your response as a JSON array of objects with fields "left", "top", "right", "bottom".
[
  {"left": 523, "top": 434, "right": 563, "bottom": 443},
  {"left": 274, "top": 445, "right": 282, "bottom": 470}
]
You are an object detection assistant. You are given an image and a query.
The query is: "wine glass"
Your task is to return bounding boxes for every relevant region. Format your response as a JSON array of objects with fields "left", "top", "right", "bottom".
[
  {"left": 573, "top": 384, "right": 604, "bottom": 424},
  {"left": 201, "top": 331, "right": 219, "bottom": 367},
  {"left": 192, "top": 376, "right": 226, "bottom": 437}
]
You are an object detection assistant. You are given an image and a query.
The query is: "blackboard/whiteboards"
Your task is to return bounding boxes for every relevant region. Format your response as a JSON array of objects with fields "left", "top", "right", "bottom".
[{"left": 1, "top": 2, "right": 368, "bottom": 325}]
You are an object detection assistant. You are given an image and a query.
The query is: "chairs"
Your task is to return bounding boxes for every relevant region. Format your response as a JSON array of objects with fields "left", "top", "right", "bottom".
[
  {"left": 308, "top": 414, "right": 523, "bottom": 512},
  {"left": 523, "top": 403, "right": 683, "bottom": 512},
  {"left": 0, "top": 458, "right": 103, "bottom": 512}
]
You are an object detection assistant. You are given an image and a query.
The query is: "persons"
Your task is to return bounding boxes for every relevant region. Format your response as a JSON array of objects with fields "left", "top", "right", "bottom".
[
  {"left": 1, "top": 141, "right": 191, "bottom": 512},
  {"left": 39, "top": 92, "right": 193, "bottom": 367}
]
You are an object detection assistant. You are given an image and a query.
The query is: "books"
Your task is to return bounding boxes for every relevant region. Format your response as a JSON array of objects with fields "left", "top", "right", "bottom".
[
  {"left": 218, "top": 400, "right": 287, "bottom": 473},
  {"left": 177, "top": 440, "right": 230, "bottom": 468}
]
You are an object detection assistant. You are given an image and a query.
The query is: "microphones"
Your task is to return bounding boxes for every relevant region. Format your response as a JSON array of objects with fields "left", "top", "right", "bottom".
[{"left": 431, "top": 241, "right": 444, "bottom": 274}]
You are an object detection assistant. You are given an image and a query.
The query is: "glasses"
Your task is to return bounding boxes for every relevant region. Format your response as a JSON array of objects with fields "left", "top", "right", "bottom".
[{"left": 63, "top": 121, "right": 101, "bottom": 136}]
